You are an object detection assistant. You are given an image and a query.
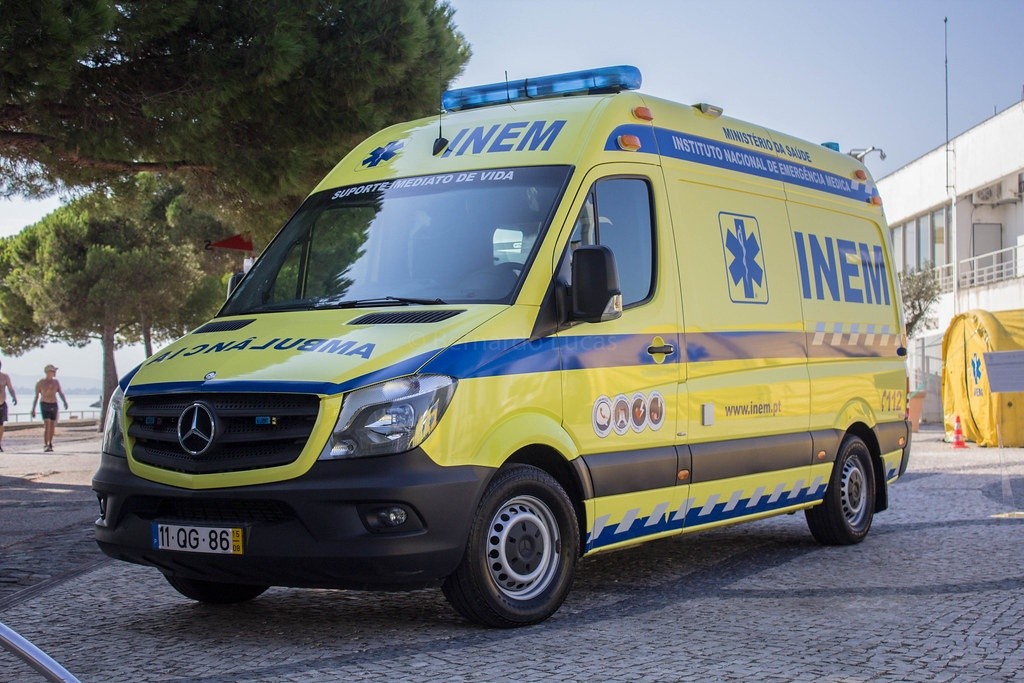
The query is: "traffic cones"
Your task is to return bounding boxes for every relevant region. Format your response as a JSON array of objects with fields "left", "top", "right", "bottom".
[{"left": 949, "top": 415, "right": 970, "bottom": 450}]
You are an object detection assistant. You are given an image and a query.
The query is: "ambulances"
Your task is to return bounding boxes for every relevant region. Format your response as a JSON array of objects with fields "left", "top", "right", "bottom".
[{"left": 91, "top": 65, "right": 914, "bottom": 630}]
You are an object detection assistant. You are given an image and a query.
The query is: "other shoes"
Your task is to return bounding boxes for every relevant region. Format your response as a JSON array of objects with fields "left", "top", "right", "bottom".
[
  {"left": 49, "top": 445, "right": 53, "bottom": 451},
  {"left": 44, "top": 446, "right": 49, "bottom": 452},
  {"left": 0, "top": 448, "right": 4, "bottom": 452}
]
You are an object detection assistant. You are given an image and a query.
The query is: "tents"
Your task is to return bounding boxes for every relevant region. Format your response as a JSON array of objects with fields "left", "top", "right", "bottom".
[{"left": 941, "top": 309, "right": 1024, "bottom": 448}]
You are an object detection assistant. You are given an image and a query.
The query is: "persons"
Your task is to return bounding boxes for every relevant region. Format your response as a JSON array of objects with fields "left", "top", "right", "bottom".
[
  {"left": 0, "top": 359, "right": 17, "bottom": 454},
  {"left": 31, "top": 366, "right": 68, "bottom": 452}
]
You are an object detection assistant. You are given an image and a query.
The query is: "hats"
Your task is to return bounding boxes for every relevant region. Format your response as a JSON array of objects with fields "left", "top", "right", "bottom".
[{"left": 45, "top": 365, "right": 58, "bottom": 372}]
